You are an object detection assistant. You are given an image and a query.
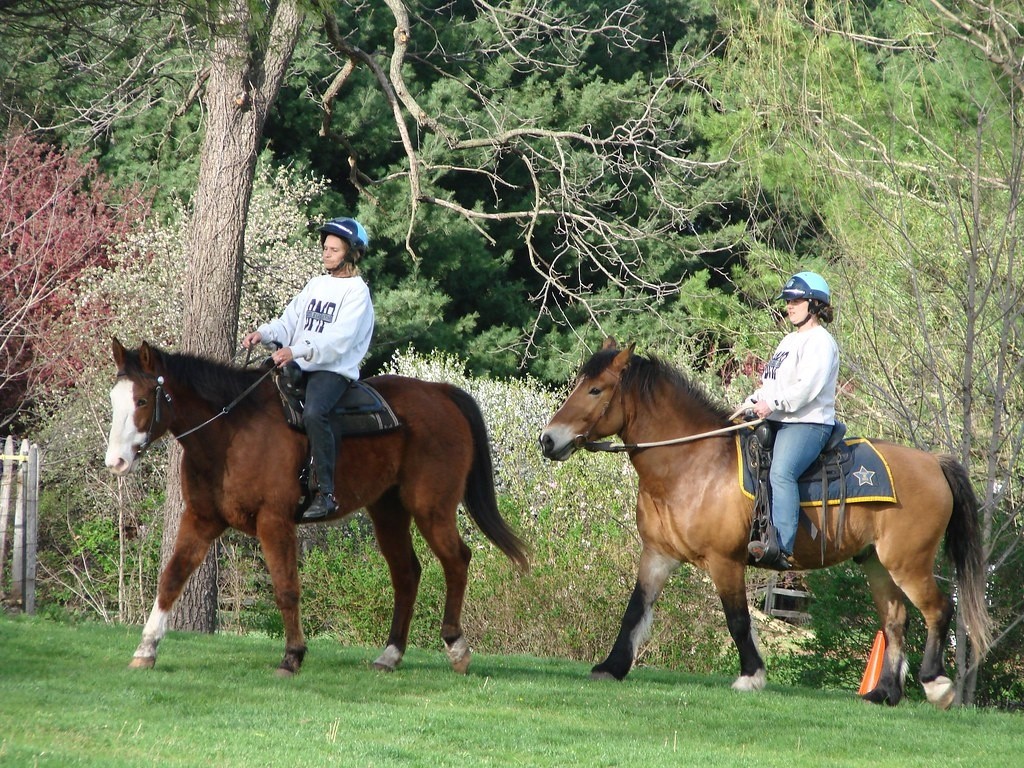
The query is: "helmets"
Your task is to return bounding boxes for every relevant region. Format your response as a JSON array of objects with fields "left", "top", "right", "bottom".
[
  {"left": 776, "top": 272, "right": 831, "bottom": 305},
  {"left": 316, "top": 217, "right": 368, "bottom": 250}
]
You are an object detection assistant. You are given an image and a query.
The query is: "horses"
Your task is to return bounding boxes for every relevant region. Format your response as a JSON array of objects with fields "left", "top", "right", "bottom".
[
  {"left": 101, "top": 331, "right": 532, "bottom": 676},
  {"left": 538, "top": 334, "right": 1002, "bottom": 711}
]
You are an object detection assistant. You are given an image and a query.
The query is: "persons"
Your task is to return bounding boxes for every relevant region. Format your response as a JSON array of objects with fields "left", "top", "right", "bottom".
[
  {"left": 734, "top": 272, "right": 840, "bottom": 564},
  {"left": 243, "top": 217, "right": 374, "bottom": 518}
]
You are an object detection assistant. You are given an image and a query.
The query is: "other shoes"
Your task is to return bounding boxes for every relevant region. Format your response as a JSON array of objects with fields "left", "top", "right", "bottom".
[{"left": 748, "top": 540, "right": 790, "bottom": 566}]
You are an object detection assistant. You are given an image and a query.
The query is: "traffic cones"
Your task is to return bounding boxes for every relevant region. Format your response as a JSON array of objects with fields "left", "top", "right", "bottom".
[{"left": 856, "top": 629, "right": 886, "bottom": 695}]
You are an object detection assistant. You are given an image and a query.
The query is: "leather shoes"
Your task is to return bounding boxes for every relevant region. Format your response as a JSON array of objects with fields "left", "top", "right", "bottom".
[{"left": 302, "top": 495, "right": 337, "bottom": 517}]
